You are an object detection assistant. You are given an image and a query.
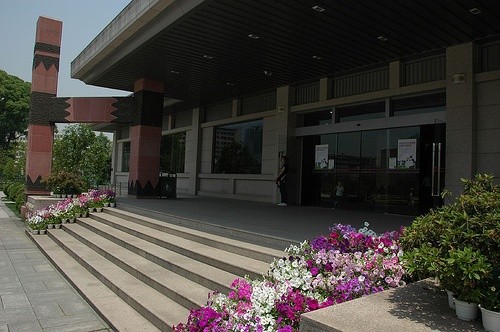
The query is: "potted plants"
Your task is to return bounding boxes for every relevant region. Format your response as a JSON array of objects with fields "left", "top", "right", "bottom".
[
  {"left": 52, "top": 188, "right": 82, "bottom": 198},
  {"left": 397, "top": 172, "right": 499, "bottom": 332}
]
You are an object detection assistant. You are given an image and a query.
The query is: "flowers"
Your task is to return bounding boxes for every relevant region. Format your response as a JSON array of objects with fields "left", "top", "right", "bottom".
[
  {"left": 25, "top": 189, "right": 118, "bottom": 230},
  {"left": 170, "top": 221, "right": 409, "bottom": 332}
]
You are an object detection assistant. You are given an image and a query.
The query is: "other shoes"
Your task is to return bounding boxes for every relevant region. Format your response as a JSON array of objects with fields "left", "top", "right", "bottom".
[{"left": 276, "top": 203, "right": 287, "bottom": 206}]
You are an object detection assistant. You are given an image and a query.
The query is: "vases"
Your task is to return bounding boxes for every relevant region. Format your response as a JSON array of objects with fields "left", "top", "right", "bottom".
[{"left": 25, "top": 203, "right": 116, "bottom": 235}]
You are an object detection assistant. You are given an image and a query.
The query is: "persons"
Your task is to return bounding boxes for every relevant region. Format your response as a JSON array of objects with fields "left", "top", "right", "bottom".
[
  {"left": 275, "top": 156, "right": 290, "bottom": 206},
  {"left": 335, "top": 181, "right": 345, "bottom": 207}
]
